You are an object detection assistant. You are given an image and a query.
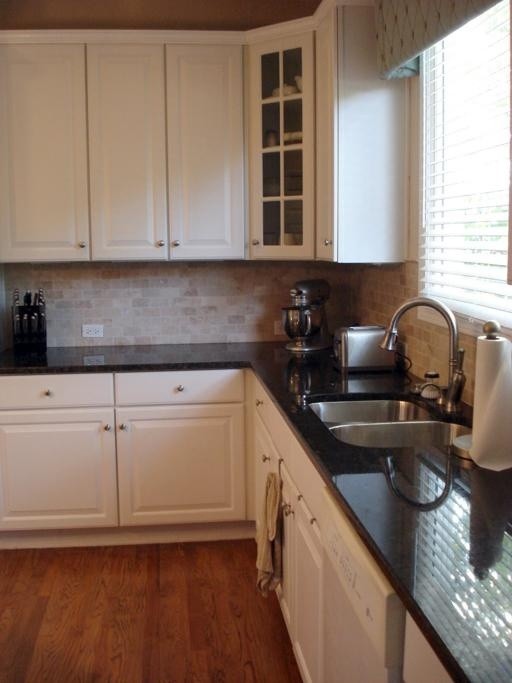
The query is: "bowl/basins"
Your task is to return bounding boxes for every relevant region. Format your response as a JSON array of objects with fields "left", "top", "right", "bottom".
[
  {"left": 272, "top": 84, "right": 297, "bottom": 95},
  {"left": 292, "top": 74, "right": 302, "bottom": 91},
  {"left": 282, "top": 130, "right": 302, "bottom": 143}
]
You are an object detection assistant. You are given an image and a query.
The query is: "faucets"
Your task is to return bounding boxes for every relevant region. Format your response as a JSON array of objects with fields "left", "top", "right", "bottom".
[{"left": 379, "top": 296, "right": 467, "bottom": 415}]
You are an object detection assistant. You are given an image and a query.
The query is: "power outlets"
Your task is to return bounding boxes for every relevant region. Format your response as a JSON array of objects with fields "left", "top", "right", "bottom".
[
  {"left": 273, "top": 319, "right": 287, "bottom": 336},
  {"left": 81, "top": 324, "right": 104, "bottom": 338}
]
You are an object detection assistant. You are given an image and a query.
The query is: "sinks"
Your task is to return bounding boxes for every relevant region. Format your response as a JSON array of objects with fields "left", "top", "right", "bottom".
[
  {"left": 305, "top": 400, "right": 440, "bottom": 421},
  {"left": 329, "top": 421, "right": 472, "bottom": 449}
]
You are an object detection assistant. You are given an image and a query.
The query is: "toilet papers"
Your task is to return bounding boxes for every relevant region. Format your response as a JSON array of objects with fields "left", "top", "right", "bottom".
[{"left": 469, "top": 335, "right": 512, "bottom": 473}]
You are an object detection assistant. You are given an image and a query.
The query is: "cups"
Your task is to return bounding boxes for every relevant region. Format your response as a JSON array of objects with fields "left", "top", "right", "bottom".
[{"left": 263, "top": 229, "right": 303, "bottom": 246}]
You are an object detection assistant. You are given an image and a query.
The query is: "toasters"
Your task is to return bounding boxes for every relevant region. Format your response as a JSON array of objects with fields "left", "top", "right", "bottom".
[{"left": 331, "top": 324, "right": 400, "bottom": 374}]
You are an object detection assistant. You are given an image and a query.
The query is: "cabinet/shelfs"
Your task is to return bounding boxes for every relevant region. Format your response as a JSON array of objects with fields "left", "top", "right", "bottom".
[
  {"left": 85, "top": 43, "right": 246, "bottom": 263},
  {"left": 251, "top": 376, "right": 325, "bottom": 683},
  {"left": 315, "top": 8, "right": 409, "bottom": 263},
  {"left": 0, "top": 369, "right": 246, "bottom": 530},
  {"left": 245, "top": 31, "right": 314, "bottom": 259},
  {"left": 0, "top": 45, "right": 91, "bottom": 264}
]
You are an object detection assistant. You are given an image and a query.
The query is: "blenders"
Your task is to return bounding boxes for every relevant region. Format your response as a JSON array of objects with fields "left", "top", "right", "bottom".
[
  {"left": 283, "top": 279, "right": 335, "bottom": 350},
  {"left": 286, "top": 351, "right": 328, "bottom": 415}
]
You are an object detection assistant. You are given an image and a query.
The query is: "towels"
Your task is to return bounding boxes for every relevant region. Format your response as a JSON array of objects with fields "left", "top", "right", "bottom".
[{"left": 255, "top": 472, "right": 282, "bottom": 598}]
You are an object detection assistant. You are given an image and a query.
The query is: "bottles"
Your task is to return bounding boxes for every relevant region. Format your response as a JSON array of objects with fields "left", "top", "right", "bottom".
[
  {"left": 266, "top": 128, "right": 276, "bottom": 147},
  {"left": 424, "top": 371, "right": 440, "bottom": 398}
]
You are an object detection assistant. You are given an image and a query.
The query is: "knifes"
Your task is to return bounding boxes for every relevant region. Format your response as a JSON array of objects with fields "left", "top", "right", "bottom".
[{"left": 11, "top": 286, "right": 47, "bottom": 333}]
